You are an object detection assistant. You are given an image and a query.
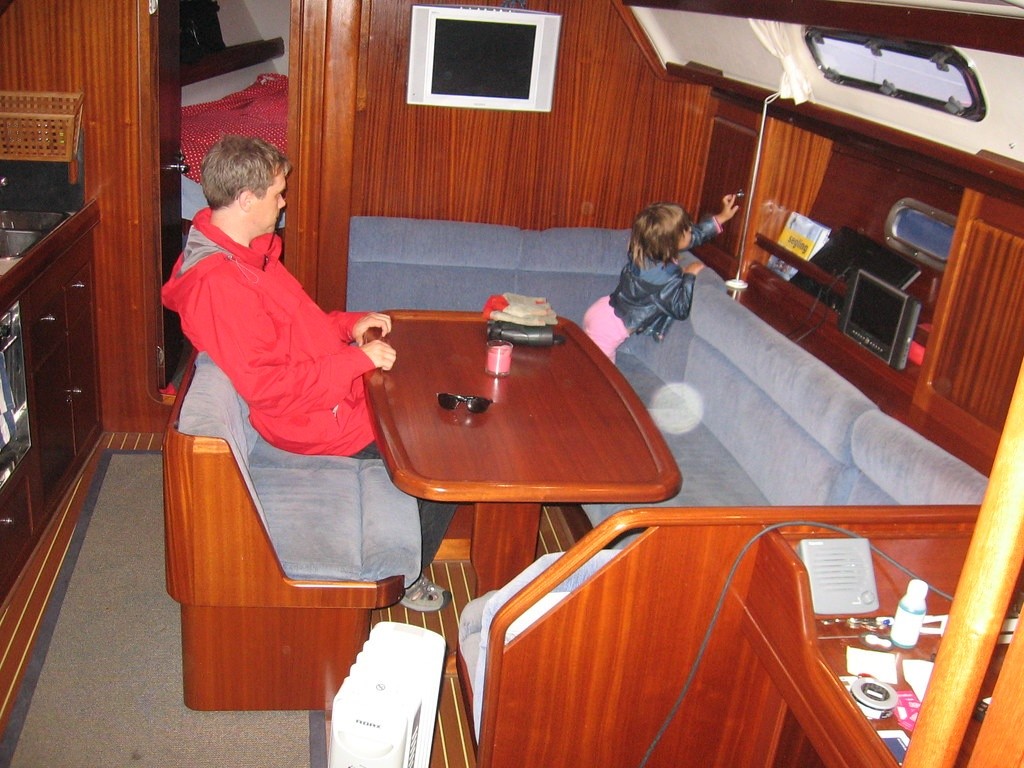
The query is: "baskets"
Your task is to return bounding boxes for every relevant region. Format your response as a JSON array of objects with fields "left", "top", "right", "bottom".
[{"left": 0, "top": 90, "right": 85, "bottom": 162}]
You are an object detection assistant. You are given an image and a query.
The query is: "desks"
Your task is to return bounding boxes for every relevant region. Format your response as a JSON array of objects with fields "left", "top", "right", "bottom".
[{"left": 717, "top": 523, "right": 1024, "bottom": 768}]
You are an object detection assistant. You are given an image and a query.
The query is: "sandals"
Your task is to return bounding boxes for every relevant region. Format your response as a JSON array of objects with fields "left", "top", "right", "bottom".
[{"left": 399, "top": 572, "right": 452, "bottom": 611}]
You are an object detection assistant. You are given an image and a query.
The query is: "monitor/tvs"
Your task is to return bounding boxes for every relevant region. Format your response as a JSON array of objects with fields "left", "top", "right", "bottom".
[
  {"left": 407, "top": 5, "right": 562, "bottom": 113},
  {"left": 839, "top": 268, "right": 922, "bottom": 371}
]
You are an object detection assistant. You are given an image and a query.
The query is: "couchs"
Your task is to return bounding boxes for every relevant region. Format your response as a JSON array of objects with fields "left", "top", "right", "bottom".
[{"left": 347, "top": 215, "right": 996, "bottom": 744}]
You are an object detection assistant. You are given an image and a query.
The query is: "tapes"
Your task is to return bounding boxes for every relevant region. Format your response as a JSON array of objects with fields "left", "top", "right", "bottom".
[{"left": 851, "top": 677, "right": 898, "bottom": 719}]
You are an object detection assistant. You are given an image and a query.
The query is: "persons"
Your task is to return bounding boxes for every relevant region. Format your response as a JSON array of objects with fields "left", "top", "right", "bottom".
[
  {"left": 584, "top": 194, "right": 738, "bottom": 364},
  {"left": 160, "top": 135, "right": 457, "bottom": 612}
]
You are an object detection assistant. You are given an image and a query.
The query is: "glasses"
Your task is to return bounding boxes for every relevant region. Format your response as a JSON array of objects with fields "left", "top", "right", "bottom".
[{"left": 436, "top": 392, "right": 493, "bottom": 414}]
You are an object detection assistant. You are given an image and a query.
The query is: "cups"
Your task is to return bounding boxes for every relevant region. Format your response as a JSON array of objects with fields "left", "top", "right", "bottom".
[{"left": 484, "top": 340, "right": 513, "bottom": 377}]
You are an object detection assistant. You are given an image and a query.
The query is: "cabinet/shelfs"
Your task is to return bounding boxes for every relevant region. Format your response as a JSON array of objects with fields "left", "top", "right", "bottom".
[
  {"left": 907, "top": 158, "right": 1024, "bottom": 479},
  {"left": 697, "top": 84, "right": 760, "bottom": 285},
  {"left": 21, "top": 232, "right": 102, "bottom": 510}
]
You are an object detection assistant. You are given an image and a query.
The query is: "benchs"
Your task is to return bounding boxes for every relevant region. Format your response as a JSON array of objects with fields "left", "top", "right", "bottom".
[{"left": 163, "top": 349, "right": 421, "bottom": 709}]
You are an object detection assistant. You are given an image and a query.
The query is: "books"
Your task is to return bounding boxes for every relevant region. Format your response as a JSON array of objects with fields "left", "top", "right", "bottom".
[{"left": 779, "top": 211, "right": 831, "bottom": 259}]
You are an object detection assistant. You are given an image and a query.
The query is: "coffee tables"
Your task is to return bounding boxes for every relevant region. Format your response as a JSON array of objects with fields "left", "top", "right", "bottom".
[{"left": 359, "top": 309, "right": 682, "bottom": 584}]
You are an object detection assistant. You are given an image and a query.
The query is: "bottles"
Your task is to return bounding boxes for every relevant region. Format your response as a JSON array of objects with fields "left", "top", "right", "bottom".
[{"left": 890, "top": 579, "right": 929, "bottom": 650}]
